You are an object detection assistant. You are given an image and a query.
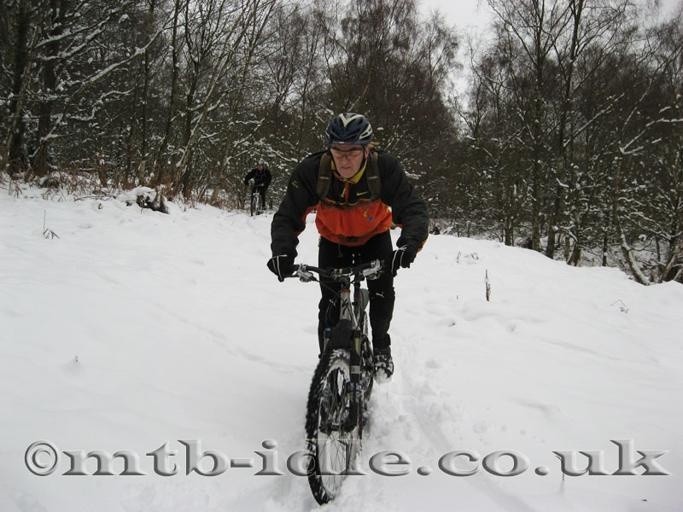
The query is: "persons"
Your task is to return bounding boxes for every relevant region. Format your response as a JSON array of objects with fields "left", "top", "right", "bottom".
[
  {"left": 269, "top": 112, "right": 431, "bottom": 435},
  {"left": 243, "top": 159, "right": 271, "bottom": 210}
]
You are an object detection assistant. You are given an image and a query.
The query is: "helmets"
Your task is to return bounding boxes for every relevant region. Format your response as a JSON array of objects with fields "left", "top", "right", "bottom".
[{"left": 323, "top": 112, "right": 376, "bottom": 146}]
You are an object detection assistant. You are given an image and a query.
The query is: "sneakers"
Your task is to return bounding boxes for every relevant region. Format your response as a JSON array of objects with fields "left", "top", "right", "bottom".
[{"left": 372, "top": 344, "right": 394, "bottom": 385}]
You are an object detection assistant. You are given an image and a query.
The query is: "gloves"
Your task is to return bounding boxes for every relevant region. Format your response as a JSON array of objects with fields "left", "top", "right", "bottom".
[
  {"left": 265, "top": 244, "right": 299, "bottom": 283},
  {"left": 383, "top": 244, "right": 414, "bottom": 278}
]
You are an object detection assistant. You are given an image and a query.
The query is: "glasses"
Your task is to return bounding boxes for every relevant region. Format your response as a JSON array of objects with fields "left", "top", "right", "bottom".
[{"left": 328, "top": 147, "right": 365, "bottom": 158}]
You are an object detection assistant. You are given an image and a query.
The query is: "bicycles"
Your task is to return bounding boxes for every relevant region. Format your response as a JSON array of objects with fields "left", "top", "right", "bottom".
[
  {"left": 287, "top": 259, "right": 388, "bottom": 505},
  {"left": 248, "top": 185, "right": 264, "bottom": 216}
]
